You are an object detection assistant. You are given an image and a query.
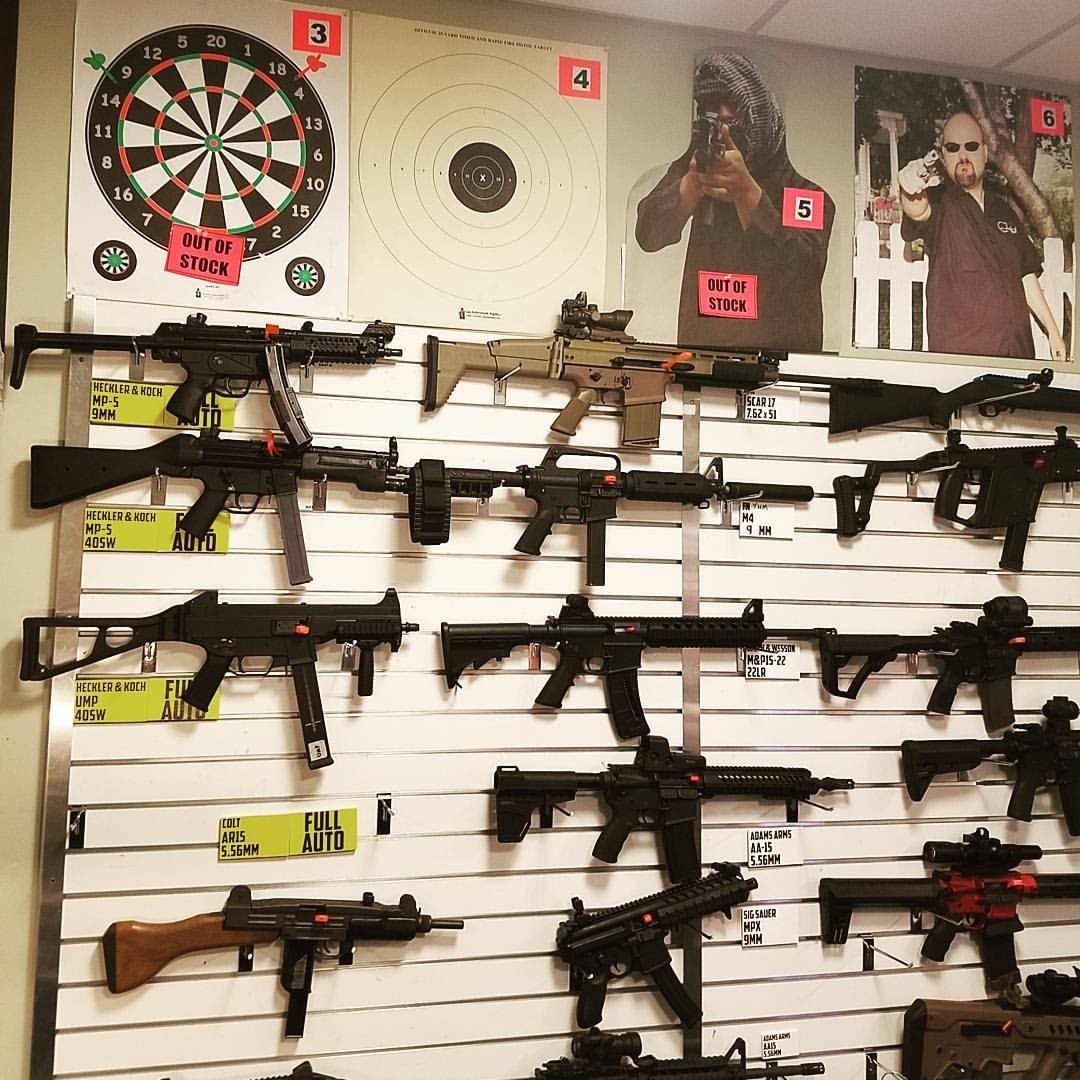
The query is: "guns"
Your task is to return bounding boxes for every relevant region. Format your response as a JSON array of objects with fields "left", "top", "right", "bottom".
[
  {"left": 902, "top": 695, "right": 1080, "bottom": 839},
  {"left": 827, "top": 366, "right": 1080, "bottom": 438},
  {"left": 689, "top": 92, "right": 728, "bottom": 228},
  {"left": 554, "top": 860, "right": 759, "bottom": 1029},
  {"left": 384, "top": 455, "right": 815, "bottom": 588},
  {"left": 831, "top": 423, "right": 1080, "bottom": 574},
  {"left": 493, "top": 734, "right": 858, "bottom": 886},
  {"left": 817, "top": 594, "right": 1080, "bottom": 734},
  {"left": 440, "top": 592, "right": 838, "bottom": 739},
  {"left": 17, "top": 586, "right": 418, "bottom": 770},
  {"left": 922, "top": 148, "right": 951, "bottom": 190},
  {"left": 495, "top": 1027, "right": 827, "bottom": 1079},
  {"left": 419, "top": 287, "right": 886, "bottom": 449},
  {"left": 29, "top": 421, "right": 399, "bottom": 587},
  {"left": 103, "top": 884, "right": 467, "bottom": 1044},
  {"left": 157, "top": 1060, "right": 348, "bottom": 1080},
  {"left": 7, "top": 313, "right": 404, "bottom": 449},
  {"left": 900, "top": 963, "right": 1080, "bottom": 1080},
  {"left": 816, "top": 826, "right": 1080, "bottom": 994}
]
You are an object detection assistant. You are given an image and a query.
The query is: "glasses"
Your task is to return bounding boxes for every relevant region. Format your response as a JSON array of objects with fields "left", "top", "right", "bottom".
[{"left": 943, "top": 141, "right": 984, "bottom": 152}]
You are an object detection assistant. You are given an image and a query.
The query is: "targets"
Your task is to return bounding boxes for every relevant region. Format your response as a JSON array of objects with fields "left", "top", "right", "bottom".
[
  {"left": 72, "top": 20, "right": 338, "bottom": 270},
  {"left": 353, "top": 44, "right": 607, "bottom": 311}
]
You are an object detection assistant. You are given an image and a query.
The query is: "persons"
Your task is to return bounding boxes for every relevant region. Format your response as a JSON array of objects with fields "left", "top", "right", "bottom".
[
  {"left": 634, "top": 49, "right": 837, "bottom": 352},
  {"left": 897, "top": 109, "right": 1067, "bottom": 363}
]
